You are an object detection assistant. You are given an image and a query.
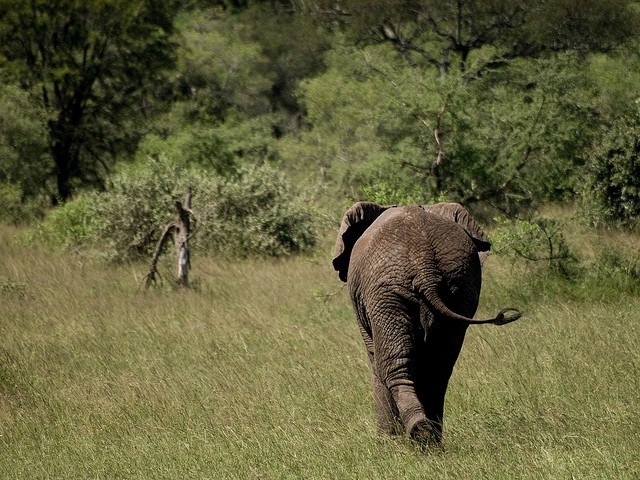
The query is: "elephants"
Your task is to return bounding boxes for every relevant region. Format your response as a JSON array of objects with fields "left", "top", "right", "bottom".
[{"left": 330, "top": 198, "right": 526, "bottom": 454}]
